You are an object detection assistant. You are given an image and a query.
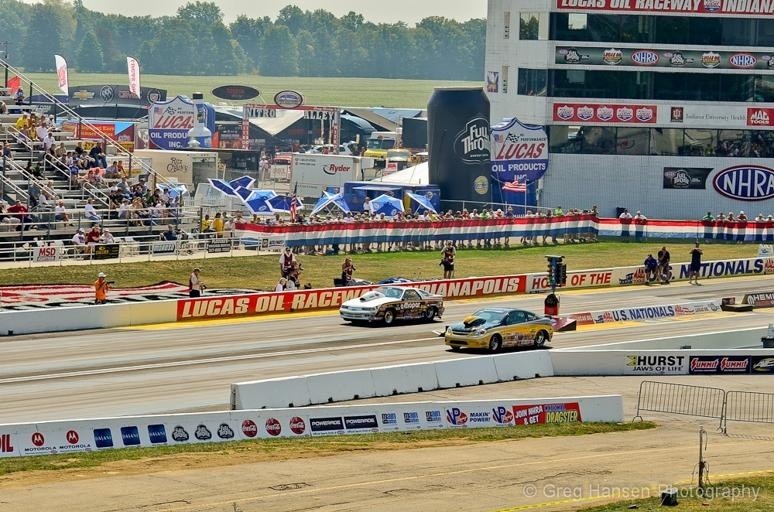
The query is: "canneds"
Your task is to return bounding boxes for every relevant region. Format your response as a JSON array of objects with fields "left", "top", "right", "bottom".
[{"left": 427, "top": 86, "right": 491, "bottom": 214}]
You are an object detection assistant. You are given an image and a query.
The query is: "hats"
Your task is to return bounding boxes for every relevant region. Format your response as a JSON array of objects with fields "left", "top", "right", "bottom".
[
  {"left": 0, "top": 86, "right": 192, "bottom": 234},
  {"left": 205, "top": 206, "right": 772, "bottom": 221},
  {"left": 98, "top": 271, "right": 106, "bottom": 278},
  {"left": 192, "top": 268, "right": 201, "bottom": 273}
]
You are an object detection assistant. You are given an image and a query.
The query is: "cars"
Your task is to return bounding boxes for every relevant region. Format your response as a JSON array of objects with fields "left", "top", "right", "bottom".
[{"left": 305, "top": 145, "right": 352, "bottom": 155}]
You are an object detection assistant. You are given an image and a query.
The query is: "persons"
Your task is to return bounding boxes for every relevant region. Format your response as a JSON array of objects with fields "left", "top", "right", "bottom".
[
  {"left": 95, "top": 272, "right": 110, "bottom": 304},
  {"left": 619, "top": 209, "right": 633, "bottom": 237},
  {"left": 0, "top": 83, "right": 181, "bottom": 259},
  {"left": 278, "top": 247, "right": 299, "bottom": 290},
  {"left": 634, "top": 210, "right": 647, "bottom": 241},
  {"left": 658, "top": 246, "right": 671, "bottom": 285},
  {"left": 257, "top": 138, "right": 339, "bottom": 180},
  {"left": 688, "top": 242, "right": 703, "bottom": 286},
  {"left": 701, "top": 131, "right": 774, "bottom": 157},
  {"left": 440, "top": 240, "right": 456, "bottom": 279},
  {"left": 703, "top": 211, "right": 774, "bottom": 243},
  {"left": 644, "top": 254, "right": 657, "bottom": 285},
  {"left": 342, "top": 257, "right": 353, "bottom": 286},
  {"left": 189, "top": 268, "right": 205, "bottom": 297}
]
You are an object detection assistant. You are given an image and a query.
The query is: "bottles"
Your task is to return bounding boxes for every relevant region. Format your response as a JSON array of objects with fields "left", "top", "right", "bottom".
[{"left": 187, "top": 92, "right": 212, "bottom": 148}]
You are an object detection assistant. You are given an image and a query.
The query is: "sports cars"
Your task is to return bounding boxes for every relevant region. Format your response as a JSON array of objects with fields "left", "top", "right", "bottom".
[
  {"left": 339, "top": 286, "right": 444, "bottom": 324},
  {"left": 445, "top": 308, "right": 554, "bottom": 351}
]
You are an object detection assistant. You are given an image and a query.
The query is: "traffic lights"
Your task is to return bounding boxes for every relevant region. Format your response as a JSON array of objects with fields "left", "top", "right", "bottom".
[{"left": 545, "top": 254, "right": 566, "bottom": 286}]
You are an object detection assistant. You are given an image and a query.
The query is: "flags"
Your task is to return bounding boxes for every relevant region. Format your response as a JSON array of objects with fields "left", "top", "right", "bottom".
[
  {"left": 290, "top": 181, "right": 298, "bottom": 220},
  {"left": 502, "top": 175, "right": 527, "bottom": 191}
]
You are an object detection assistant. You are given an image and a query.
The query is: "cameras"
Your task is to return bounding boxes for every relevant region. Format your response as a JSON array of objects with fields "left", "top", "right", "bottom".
[{"left": 105, "top": 281, "right": 115, "bottom": 285}]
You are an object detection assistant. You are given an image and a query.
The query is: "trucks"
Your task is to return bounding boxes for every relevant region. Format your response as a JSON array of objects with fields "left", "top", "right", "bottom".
[{"left": 364, "top": 132, "right": 402, "bottom": 167}]
